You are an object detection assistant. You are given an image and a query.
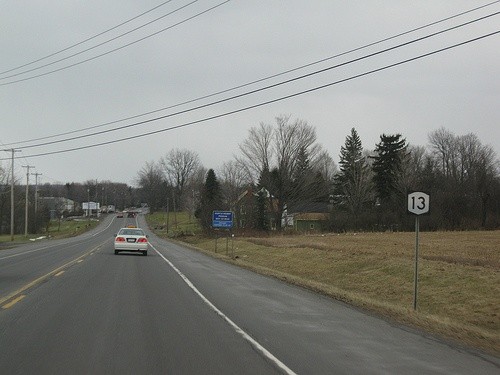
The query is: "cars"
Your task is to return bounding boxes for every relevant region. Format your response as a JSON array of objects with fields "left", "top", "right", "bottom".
[{"left": 113, "top": 225, "right": 149, "bottom": 255}]
